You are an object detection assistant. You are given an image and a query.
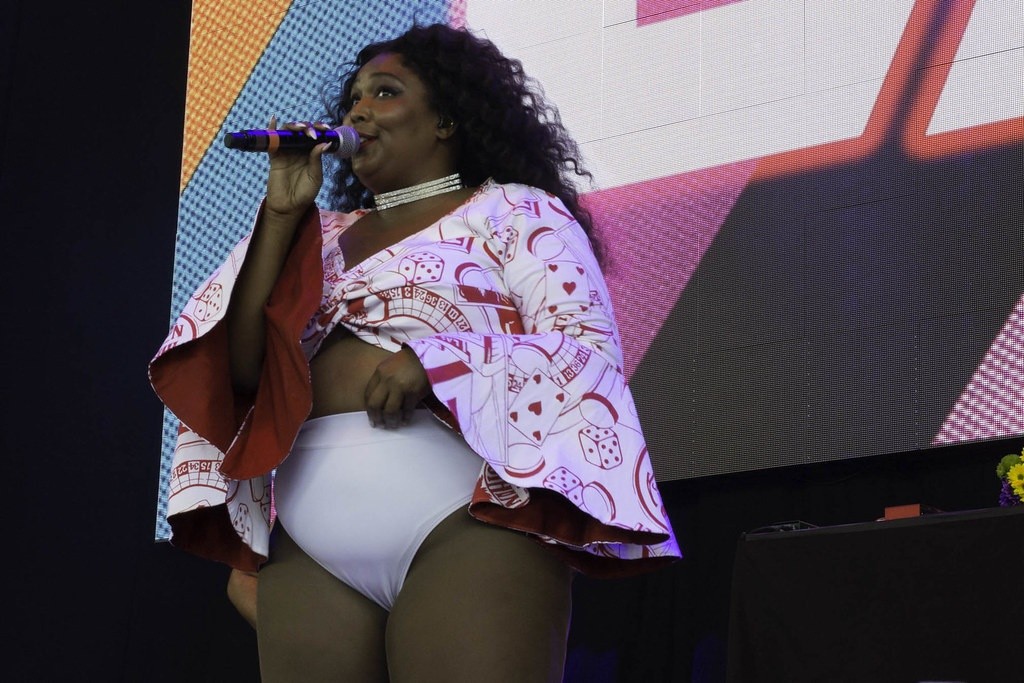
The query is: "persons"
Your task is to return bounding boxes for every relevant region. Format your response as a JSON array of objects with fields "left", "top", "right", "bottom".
[{"left": 146, "top": 10, "right": 683, "bottom": 683}]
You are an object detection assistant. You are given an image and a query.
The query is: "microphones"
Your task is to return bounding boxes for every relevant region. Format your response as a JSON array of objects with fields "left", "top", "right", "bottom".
[{"left": 225, "top": 126, "right": 361, "bottom": 157}]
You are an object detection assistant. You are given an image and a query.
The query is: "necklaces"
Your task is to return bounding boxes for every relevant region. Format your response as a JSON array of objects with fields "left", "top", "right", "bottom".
[{"left": 372, "top": 173, "right": 464, "bottom": 212}]
